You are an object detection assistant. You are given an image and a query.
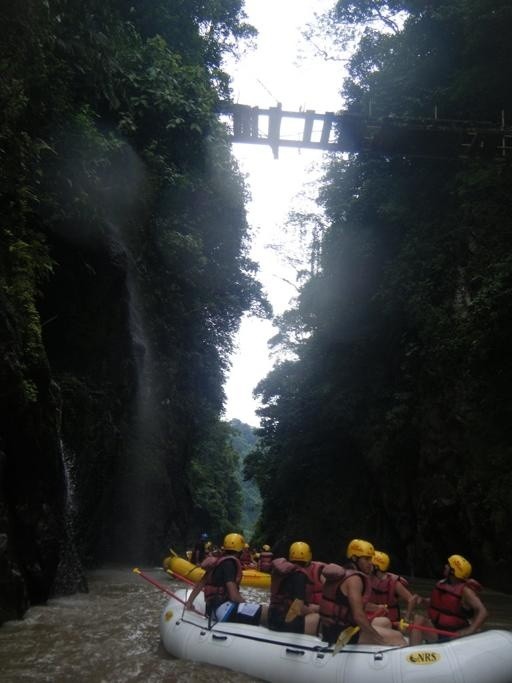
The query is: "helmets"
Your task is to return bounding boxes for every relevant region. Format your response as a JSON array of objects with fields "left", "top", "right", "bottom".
[
  {"left": 289, "top": 542, "right": 312, "bottom": 566},
  {"left": 347, "top": 539, "right": 374, "bottom": 559},
  {"left": 372, "top": 551, "right": 390, "bottom": 572},
  {"left": 224, "top": 533, "right": 247, "bottom": 552},
  {"left": 448, "top": 554, "right": 472, "bottom": 579}
]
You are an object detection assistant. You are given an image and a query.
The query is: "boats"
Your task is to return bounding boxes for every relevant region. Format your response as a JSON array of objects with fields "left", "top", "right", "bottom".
[
  {"left": 162, "top": 556, "right": 273, "bottom": 587},
  {"left": 159, "top": 587, "right": 512, "bottom": 683}
]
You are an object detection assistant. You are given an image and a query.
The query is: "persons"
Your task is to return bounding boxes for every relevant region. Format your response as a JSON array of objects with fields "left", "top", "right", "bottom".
[
  {"left": 307, "top": 559, "right": 328, "bottom": 604},
  {"left": 320, "top": 539, "right": 406, "bottom": 647},
  {"left": 191, "top": 531, "right": 276, "bottom": 578},
  {"left": 183, "top": 533, "right": 270, "bottom": 628},
  {"left": 408, "top": 554, "right": 487, "bottom": 644},
  {"left": 368, "top": 549, "right": 414, "bottom": 632},
  {"left": 268, "top": 541, "right": 321, "bottom": 635}
]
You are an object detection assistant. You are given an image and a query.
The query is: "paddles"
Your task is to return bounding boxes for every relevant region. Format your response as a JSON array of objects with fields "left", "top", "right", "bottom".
[{"left": 333, "top": 604, "right": 387, "bottom": 656}]
